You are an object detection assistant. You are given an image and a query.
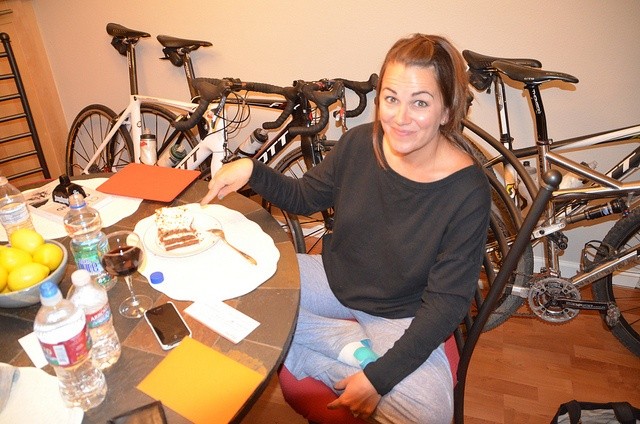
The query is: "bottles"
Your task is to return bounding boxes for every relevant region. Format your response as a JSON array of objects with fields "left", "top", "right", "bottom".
[
  {"left": 559, "top": 162, "right": 597, "bottom": 190},
  {"left": 33, "top": 281, "right": 106, "bottom": 411},
  {"left": 517, "top": 162, "right": 545, "bottom": 225},
  {"left": 0, "top": 177, "right": 36, "bottom": 244},
  {"left": 233, "top": 128, "right": 269, "bottom": 159},
  {"left": 154, "top": 144, "right": 186, "bottom": 167},
  {"left": 140, "top": 127, "right": 156, "bottom": 166},
  {"left": 66, "top": 269, "right": 118, "bottom": 368},
  {"left": 64, "top": 193, "right": 112, "bottom": 285}
]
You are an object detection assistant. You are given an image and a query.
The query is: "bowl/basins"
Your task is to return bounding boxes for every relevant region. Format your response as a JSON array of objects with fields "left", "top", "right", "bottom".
[{"left": 1, "top": 239, "right": 68, "bottom": 309}]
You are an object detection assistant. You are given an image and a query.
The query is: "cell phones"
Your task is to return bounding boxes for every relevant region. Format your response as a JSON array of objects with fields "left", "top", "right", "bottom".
[{"left": 143, "top": 300, "right": 192, "bottom": 351}]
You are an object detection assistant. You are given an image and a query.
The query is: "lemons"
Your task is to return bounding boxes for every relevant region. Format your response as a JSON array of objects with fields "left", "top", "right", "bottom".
[
  {"left": 8, "top": 264, "right": 50, "bottom": 291},
  {"left": 1, "top": 286, "right": 11, "bottom": 296},
  {"left": 10, "top": 229, "right": 44, "bottom": 254},
  {"left": 0, "top": 247, "right": 34, "bottom": 269},
  {"left": 0, "top": 267, "right": 8, "bottom": 292},
  {"left": 33, "top": 243, "right": 63, "bottom": 269}
]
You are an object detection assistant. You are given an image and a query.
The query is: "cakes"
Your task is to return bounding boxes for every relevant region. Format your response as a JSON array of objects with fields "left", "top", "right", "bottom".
[{"left": 156, "top": 207, "right": 198, "bottom": 251}]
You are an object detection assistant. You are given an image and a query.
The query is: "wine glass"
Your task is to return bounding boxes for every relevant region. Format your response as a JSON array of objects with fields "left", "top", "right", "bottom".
[{"left": 96, "top": 230, "right": 152, "bottom": 320}]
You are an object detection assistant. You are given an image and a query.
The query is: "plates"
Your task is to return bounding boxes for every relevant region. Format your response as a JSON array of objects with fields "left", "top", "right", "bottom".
[
  {"left": 0, "top": 367, "right": 85, "bottom": 424},
  {"left": 126, "top": 202, "right": 281, "bottom": 302},
  {"left": 0, "top": 177, "right": 143, "bottom": 243}
]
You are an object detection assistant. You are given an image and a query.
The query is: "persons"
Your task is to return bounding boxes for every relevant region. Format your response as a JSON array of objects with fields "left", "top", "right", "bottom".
[{"left": 200, "top": 33, "right": 493, "bottom": 424}]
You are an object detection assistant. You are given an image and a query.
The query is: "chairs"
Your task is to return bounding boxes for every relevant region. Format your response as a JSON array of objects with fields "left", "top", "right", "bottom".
[
  {"left": 367, "top": 72, "right": 561, "bottom": 424},
  {"left": 1, "top": 33, "right": 51, "bottom": 187}
]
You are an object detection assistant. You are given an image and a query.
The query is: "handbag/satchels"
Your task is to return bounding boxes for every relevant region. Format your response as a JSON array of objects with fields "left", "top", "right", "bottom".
[{"left": 549, "top": 400, "right": 640, "bottom": 423}]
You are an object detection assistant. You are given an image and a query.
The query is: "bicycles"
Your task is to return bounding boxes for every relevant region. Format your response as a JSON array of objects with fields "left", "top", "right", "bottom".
[
  {"left": 64, "top": 22, "right": 345, "bottom": 254},
  {"left": 442, "top": 49, "right": 640, "bottom": 322},
  {"left": 445, "top": 58, "right": 640, "bottom": 360},
  {"left": 106, "top": 35, "right": 380, "bottom": 257}
]
